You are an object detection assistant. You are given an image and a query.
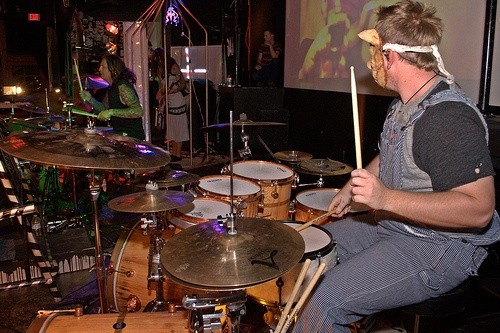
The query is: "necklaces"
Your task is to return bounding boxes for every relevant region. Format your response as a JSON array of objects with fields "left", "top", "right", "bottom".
[{"left": 404, "top": 70, "right": 440, "bottom": 105}]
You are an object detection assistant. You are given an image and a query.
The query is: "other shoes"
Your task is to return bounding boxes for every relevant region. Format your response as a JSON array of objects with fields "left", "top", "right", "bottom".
[{"left": 170, "top": 154, "right": 183, "bottom": 162}]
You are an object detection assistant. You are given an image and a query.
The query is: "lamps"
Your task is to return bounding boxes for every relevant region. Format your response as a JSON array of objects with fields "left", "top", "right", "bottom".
[
  {"left": 106, "top": 19, "right": 120, "bottom": 35},
  {"left": 106, "top": 41, "right": 120, "bottom": 55}
]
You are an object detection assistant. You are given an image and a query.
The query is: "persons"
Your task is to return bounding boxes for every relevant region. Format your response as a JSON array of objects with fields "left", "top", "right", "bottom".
[
  {"left": 290, "top": 0, "right": 500, "bottom": 333},
  {"left": 79, "top": 54, "right": 146, "bottom": 140},
  {"left": 151, "top": 48, "right": 190, "bottom": 161},
  {"left": 254, "top": 30, "right": 282, "bottom": 86}
]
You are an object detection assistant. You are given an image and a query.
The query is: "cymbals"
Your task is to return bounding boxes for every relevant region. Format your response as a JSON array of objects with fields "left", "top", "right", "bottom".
[
  {"left": 273, "top": 151, "right": 314, "bottom": 162},
  {"left": 158, "top": 215, "right": 307, "bottom": 292},
  {"left": 200, "top": 120, "right": 288, "bottom": 129},
  {"left": 295, "top": 158, "right": 355, "bottom": 176},
  {"left": 106, "top": 170, "right": 201, "bottom": 213},
  {"left": 0, "top": 132, "right": 170, "bottom": 169},
  {"left": 14, "top": 102, "right": 47, "bottom": 113}
]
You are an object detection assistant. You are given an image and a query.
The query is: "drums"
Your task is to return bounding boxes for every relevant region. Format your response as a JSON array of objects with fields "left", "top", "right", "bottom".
[
  {"left": 170, "top": 159, "right": 375, "bottom": 223},
  {"left": 25, "top": 307, "right": 235, "bottom": 333},
  {"left": 7, "top": 116, "right": 65, "bottom": 175},
  {"left": 104, "top": 213, "right": 224, "bottom": 314},
  {"left": 245, "top": 220, "right": 337, "bottom": 309}
]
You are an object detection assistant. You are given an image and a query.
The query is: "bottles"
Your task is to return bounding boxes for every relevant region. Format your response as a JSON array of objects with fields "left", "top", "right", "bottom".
[{"left": 227, "top": 75, "right": 232, "bottom": 86}]
